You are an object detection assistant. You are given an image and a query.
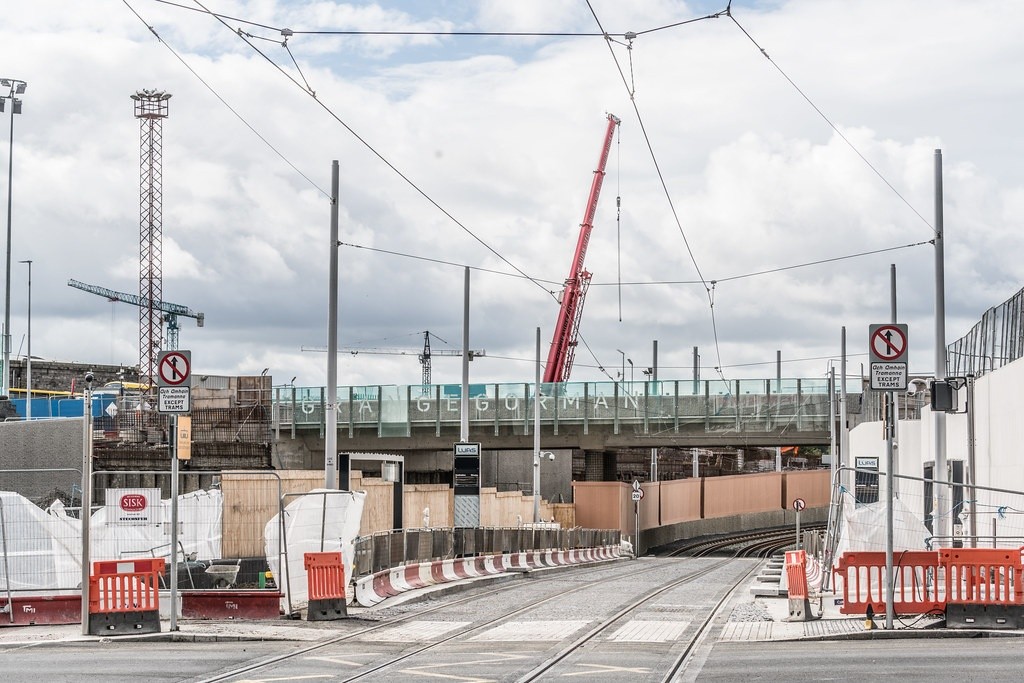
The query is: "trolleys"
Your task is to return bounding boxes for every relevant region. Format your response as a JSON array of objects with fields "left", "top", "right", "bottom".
[{"left": 204, "top": 559, "right": 241, "bottom": 590}]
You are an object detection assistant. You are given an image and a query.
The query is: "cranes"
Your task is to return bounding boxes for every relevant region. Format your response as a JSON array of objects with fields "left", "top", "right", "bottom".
[
  {"left": 67, "top": 278, "right": 205, "bottom": 411},
  {"left": 299, "top": 330, "right": 486, "bottom": 400}
]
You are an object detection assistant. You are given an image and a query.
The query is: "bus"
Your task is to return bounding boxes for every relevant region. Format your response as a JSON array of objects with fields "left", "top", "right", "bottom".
[{"left": 104, "top": 381, "right": 158, "bottom": 408}]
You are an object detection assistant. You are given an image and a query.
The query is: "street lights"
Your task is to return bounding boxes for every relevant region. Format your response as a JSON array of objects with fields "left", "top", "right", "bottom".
[
  {"left": 18, "top": 260, "right": 34, "bottom": 420},
  {"left": 617, "top": 350, "right": 624, "bottom": 395},
  {"left": 628, "top": 359, "right": 633, "bottom": 395}
]
